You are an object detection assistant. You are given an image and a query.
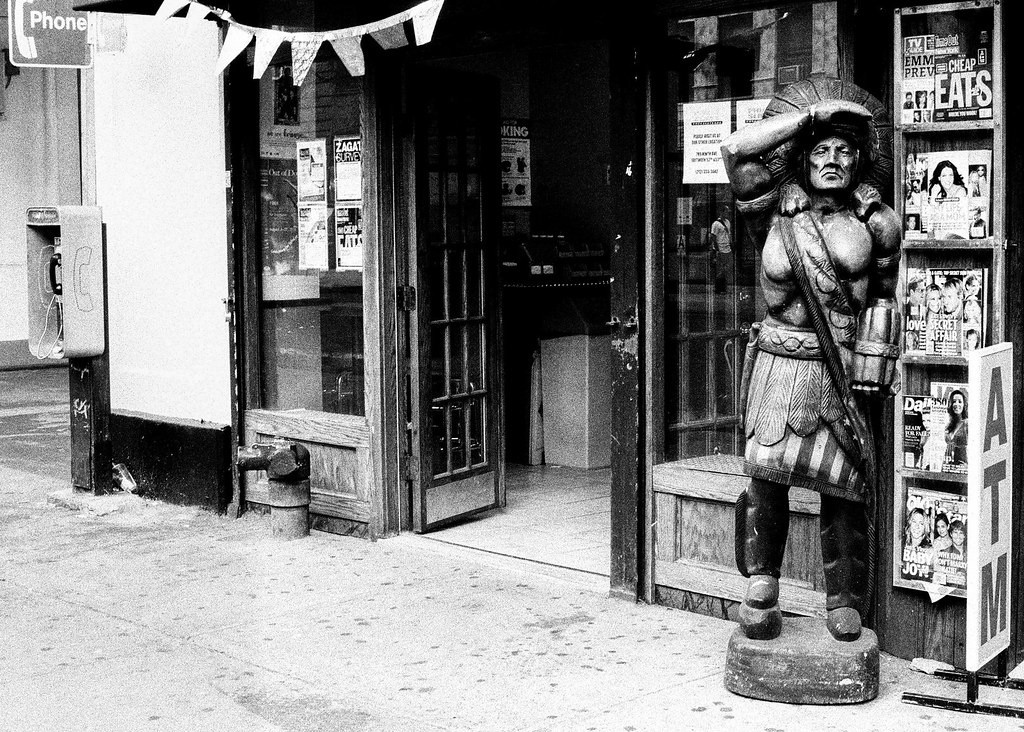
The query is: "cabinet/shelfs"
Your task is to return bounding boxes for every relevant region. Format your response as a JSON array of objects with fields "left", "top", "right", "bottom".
[{"left": 893, "top": 0, "right": 1007, "bottom": 601}]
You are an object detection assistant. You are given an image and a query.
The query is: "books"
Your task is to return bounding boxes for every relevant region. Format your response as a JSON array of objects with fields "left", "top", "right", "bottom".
[
  {"left": 902, "top": 265, "right": 988, "bottom": 355},
  {"left": 900, "top": 486, "right": 966, "bottom": 589},
  {"left": 902, "top": 34, "right": 935, "bottom": 122},
  {"left": 905, "top": 149, "right": 992, "bottom": 239},
  {"left": 902, "top": 381, "right": 974, "bottom": 474},
  {"left": 935, "top": 31, "right": 994, "bottom": 121}
]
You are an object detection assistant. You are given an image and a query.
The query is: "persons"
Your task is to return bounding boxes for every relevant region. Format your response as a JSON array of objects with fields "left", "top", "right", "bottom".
[
  {"left": 903, "top": 89, "right": 933, "bottom": 121},
  {"left": 967, "top": 163, "right": 986, "bottom": 198},
  {"left": 915, "top": 406, "right": 934, "bottom": 469},
  {"left": 929, "top": 160, "right": 968, "bottom": 204},
  {"left": 905, "top": 151, "right": 928, "bottom": 205},
  {"left": 902, "top": 506, "right": 966, "bottom": 589},
  {"left": 941, "top": 388, "right": 967, "bottom": 475},
  {"left": 905, "top": 213, "right": 919, "bottom": 230},
  {"left": 719, "top": 104, "right": 908, "bottom": 642},
  {"left": 970, "top": 208, "right": 986, "bottom": 238},
  {"left": 905, "top": 273, "right": 980, "bottom": 354},
  {"left": 710, "top": 203, "right": 737, "bottom": 293}
]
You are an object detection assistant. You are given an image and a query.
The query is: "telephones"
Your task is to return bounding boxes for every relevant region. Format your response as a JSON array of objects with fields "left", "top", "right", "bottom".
[{"left": 48, "top": 236, "right": 62, "bottom": 302}]
